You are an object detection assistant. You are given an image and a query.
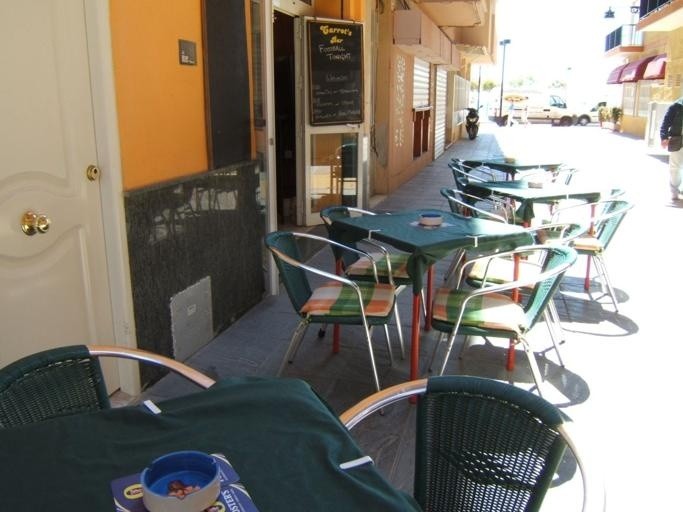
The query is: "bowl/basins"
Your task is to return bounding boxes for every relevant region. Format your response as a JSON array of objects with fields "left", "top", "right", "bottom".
[
  {"left": 140, "top": 450, "right": 221, "bottom": 511},
  {"left": 419, "top": 213, "right": 443, "bottom": 226},
  {"left": 528, "top": 182, "right": 542, "bottom": 189},
  {"left": 504, "top": 158, "right": 515, "bottom": 163}
]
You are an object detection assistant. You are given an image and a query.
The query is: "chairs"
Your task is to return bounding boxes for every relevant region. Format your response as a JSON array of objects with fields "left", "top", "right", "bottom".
[
  {"left": 450, "top": 156, "right": 585, "bottom": 224},
  {"left": 336, "top": 376, "right": 588, "bottom": 508},
  {"left": 320, "top": 205, "right": 430, "bottom": 361},
  {"left": 466, "top": 220, "right": 587, "bottom": 358},
  {"left": 264, "top": 230, "right": 397, "bottom": 415},
  {"left": 0, "top": 344, "right": 248, "bottom": 509},
  {"left": 426, "top": 245, "right": 578, "bottom": 417},
  {"left": 440, "top": 186, "right": 525, "bottom": 283},
  {"left": 533, "top": 199, "right": 629, "bottom": 322}
]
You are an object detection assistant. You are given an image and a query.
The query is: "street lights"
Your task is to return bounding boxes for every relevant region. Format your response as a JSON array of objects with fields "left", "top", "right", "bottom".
[{"left": 497, "top": 37, "right": 512, "bottom": 120}]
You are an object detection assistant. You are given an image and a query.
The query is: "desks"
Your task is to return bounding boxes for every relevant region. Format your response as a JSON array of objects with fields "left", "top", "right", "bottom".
[
  {"left": 2, "top": 378, "right": 417, "bottom": 509},
  {"left": 340, "top": 209, "right": 532, "bottom": 332}
]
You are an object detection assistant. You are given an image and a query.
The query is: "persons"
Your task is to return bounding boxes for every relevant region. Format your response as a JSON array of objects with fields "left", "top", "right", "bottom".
[{"left": 658, "top": 92, "right": 682, "bottom": 207}]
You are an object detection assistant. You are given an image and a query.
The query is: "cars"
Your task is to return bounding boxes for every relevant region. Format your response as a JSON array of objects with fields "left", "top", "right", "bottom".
[
  {"left": 548, "top": 96, "right": 599, "bottom": 125},
  {"left": 578, "top": 101, "right": 606, "bottom": 125},
  {"left": 487, "top": 102, "right": 579, "bottom": 127}
]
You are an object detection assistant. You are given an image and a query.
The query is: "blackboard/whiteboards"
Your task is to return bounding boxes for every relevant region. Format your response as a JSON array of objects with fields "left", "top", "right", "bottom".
[{"left": 307, "top": 19, "right": 365, "bottom": 127}]
